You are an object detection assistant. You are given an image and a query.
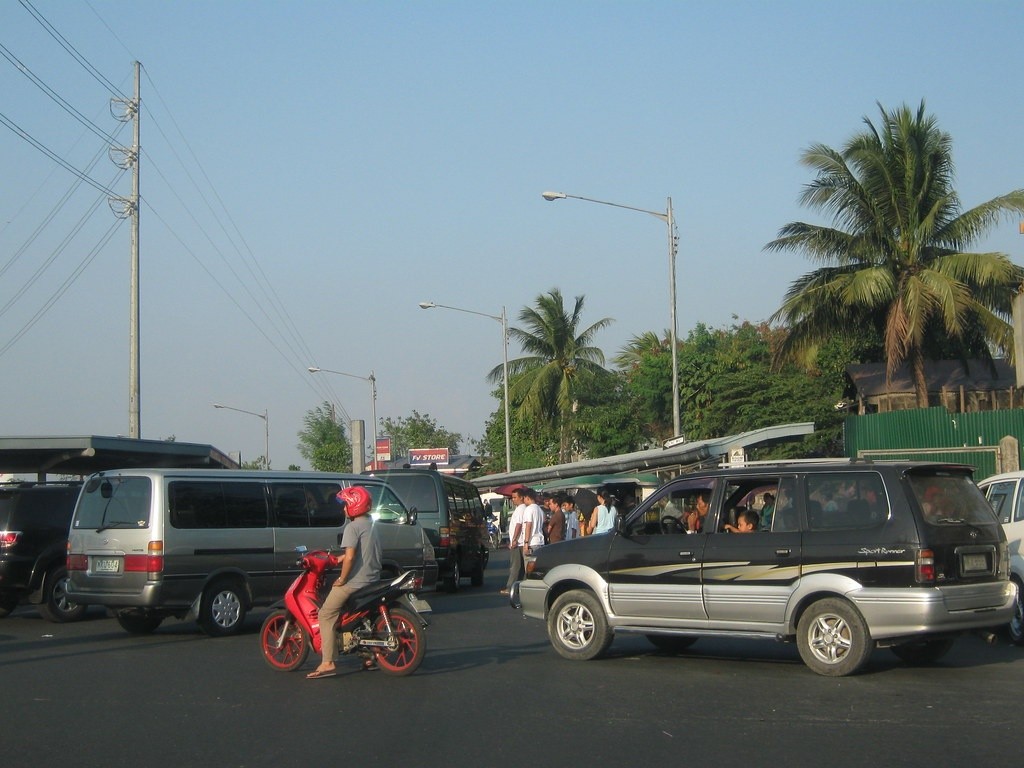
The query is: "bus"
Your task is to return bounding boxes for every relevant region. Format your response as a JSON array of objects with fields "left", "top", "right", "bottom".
[{"left": 503, "top": 473, "right": 659, "bottom": 549}]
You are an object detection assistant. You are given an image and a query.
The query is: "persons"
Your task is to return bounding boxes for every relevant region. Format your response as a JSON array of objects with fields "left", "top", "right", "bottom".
[
  {"left": 722, "top": 511, "right": 759, "bottom": 534},
  {"left": 482, "top": 486, "right": 622, "bottom": 597},
  {"left": 679, "top": 490, "right": 712, "bottom": 535},
  {"left": 751, "top": 479, "right": 887, "bottom": 532},
  {"left": 304, "top": 486, "right": 383, "bottom": 680}
]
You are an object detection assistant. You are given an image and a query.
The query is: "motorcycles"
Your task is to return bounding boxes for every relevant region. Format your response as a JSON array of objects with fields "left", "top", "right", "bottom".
[
  {"left": 258, "top": 546, "right": 432, "bottom": 679},
  {"left": 487, "top": 515, "right": 500, "bottom": 550}
]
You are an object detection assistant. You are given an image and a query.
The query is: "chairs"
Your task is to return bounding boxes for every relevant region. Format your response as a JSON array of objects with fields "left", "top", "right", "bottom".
[{"left": 730, "top": 505, "right": 745, "bottom": 532}]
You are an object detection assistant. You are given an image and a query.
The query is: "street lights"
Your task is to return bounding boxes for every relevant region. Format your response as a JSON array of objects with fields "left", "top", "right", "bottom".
[
  {"left": 542, "top": 190, "right": 681, "bottom": 443},
  {"left": 309, "top": 365, "right": 378, "bottom": 469},
  {"left": 419, "top": 301, "right": 512, "bottom": 472},
  {"left": 214, "top": 404, "right": 269, "bottom": 470}
]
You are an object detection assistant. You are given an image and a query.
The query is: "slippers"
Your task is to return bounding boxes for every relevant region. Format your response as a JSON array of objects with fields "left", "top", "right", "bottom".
[
  {"left": 306, "top": 670, "right": 336, "bottom": 678},
  {"left": 361, "top": 659, "right": 378, "bottom": 670}
]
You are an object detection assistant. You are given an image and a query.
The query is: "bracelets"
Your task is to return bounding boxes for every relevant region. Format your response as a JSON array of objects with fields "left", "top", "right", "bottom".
[
  {"left": 339, "top": 577, "right": 347, "bottom": 585},
  {"left": 525, "top": 541, "right": 528, "bottom": 543}
]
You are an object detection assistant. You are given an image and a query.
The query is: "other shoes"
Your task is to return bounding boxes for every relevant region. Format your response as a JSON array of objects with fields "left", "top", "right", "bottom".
[{"left": 501, "top": 587, "right": 511, "bottom": 594}]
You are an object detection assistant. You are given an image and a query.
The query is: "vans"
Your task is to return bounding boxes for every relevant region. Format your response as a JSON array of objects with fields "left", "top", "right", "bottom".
[
  {"left": 957, "top": 469, "right": 1024, "bottom": 646},
  {"left": 359, "top": 469, "right": 489, "bottom": 593},
  {"left": 60, "top": 469, "right": 438, "bottom": 637}
]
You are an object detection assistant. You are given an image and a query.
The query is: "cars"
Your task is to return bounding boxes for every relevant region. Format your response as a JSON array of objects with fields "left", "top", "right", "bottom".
[{"left": 480, "top": 492, "right": 518, "bottom": 545}]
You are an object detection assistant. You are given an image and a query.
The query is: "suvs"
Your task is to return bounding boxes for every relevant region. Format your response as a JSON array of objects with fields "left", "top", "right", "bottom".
[
  {"left": 509, "top": 456, "right": 1019, "bottom": 678},
  {"left": 0, "top": 480, "right": 87, "bottom": 624}
]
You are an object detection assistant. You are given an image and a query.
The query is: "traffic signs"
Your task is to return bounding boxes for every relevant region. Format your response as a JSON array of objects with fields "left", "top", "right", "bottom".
[{"left": 662, "top": 433, "right": 686, "bottom": 452}]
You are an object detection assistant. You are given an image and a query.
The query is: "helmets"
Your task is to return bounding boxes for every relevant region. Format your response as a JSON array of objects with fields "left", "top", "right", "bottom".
[{"left": 335, "top": 486, "right": 372, "bottom": 518}]
